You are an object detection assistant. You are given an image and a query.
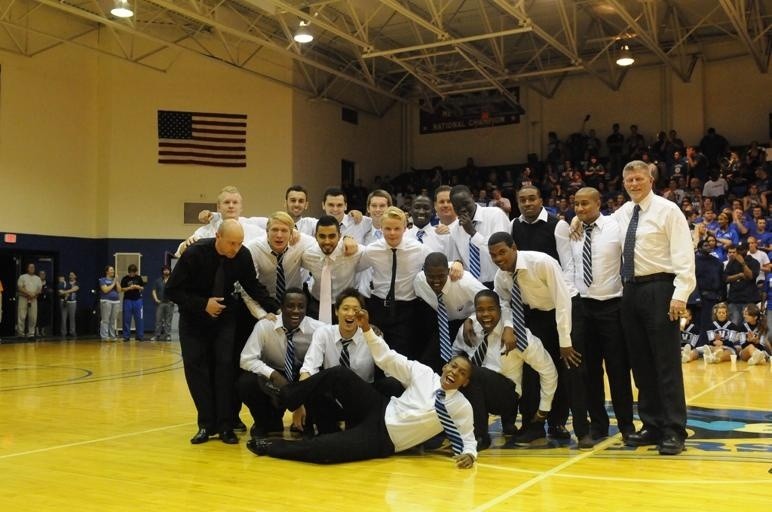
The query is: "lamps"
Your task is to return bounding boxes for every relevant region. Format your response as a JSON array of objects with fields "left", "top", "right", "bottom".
[
  {"left": 109, "top": 0, "right": 135, "bottom": 19},
  {"left": 293, "top": 17, "right": 313, "bottom": 44},
  {"left": 614, "top": 40, "right": 636, "bottom": 66}
]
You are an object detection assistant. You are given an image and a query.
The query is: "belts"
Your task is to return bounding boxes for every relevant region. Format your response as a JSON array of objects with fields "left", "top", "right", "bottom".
[{"left": 625, "top": 271, "right": 676, "bottom": 287}]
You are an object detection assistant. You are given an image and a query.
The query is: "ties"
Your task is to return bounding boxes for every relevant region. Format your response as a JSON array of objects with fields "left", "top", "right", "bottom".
[
  {"left": 212, "top": 257, "right": 225, "bottom": 305},
  {"left": 621, "top": 204, "right": 641, "bottom": 283},
  {"left": 271, "top": 246, "right": 289, "bottom": 305},
  {"left": 468, "top": 221, "right": 481, "bottom": 279},
  {"left": 470, "top": 331, "right": 489, "bottom": 368},
  {"left": 339, "top": 222, "right": 343, "bottom": 234},
  {"left": 434, "top": 388, "right": 465, "bottom": 456},
  {"left": 338, "top": 338, "right": 353, "bottom": 369},
  {"left": 434, "top": 290, "right": 453, "bottom": 364},
  {"left": 294, "top": 224, "right": 298, "bottom": 230},
  {"left": 374, "top": 230, "right": 382, "bottom": 239},
  {"left": 509, "top": 271, "right": 529, "bottom": 353},
  {"left": 416, "top": 230, "right": 425, "bottom": 244},
  {"left": 280, "top": 326, "right": 301, "bottom": 384},
  {"left": 318, "top": 257, "right": 334, "bottom": 326},
  {"left": 582, "top": 225, "right": 594, "bottom": 289},
  {"left": 385, "top": 247, "right": 398, "bottom": 313}
]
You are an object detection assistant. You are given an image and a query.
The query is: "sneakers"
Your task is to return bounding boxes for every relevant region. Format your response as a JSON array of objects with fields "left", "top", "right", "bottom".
[
  {"left": 16, "top": 335, "right": 148, "bottom": 343},
  {"left": 681, "top": 342, "right": 767, "bottom": 366}
]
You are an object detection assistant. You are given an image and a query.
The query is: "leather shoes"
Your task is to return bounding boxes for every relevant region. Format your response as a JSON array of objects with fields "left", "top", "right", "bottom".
[
  {"left": 290, "top": 423, "right": 299, "bottom": 432},
  {"left": 256, "top": 372, "right": 288, "bottom": 410},
  {"left": 657, "top": 433, "right": 685, "bottom": 455},
  {"left": 591, "top": 431, "right": 609, "bottom": 440},
  {"left": 475, "top": 434, "right": 492, "bottom": 451},
  {"left": 578, "top": 434, "right": 593, "bottom": 449},
  {"left": 501, "top": 423, "right": 519, "bottom": 435},
  {"left": 245, "top": 437, "right": 273, "bottom": 456},
  {"left": 513, "top": 422, "right": 548, "bottom": 443},
  {"left": 190, "top": 425, "right": 209, "bottom": 444},
  {"left": 216, "top": 428, "right": 239, "bottom": 444},
  {"left": 621, "top": 430, "right": 634, "bottom": 439},
  {"left": 625, "top": 425, "right": 661, "bottom": 446},
  {"left": 231, "top": 417, "right": 247, "bottom": 431},
  {"left": 547, "top": 423, "right": 572, "bottom": 439}
]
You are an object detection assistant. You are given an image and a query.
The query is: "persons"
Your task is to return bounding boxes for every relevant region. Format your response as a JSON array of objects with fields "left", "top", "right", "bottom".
[
  {"left": 56, "top": 276, "right": 71, "bottom": 306},
  {"left": 17, "top": 264, "right": 42, "bottom": 340},
  {"left": 98, "top": 265, "right": 121, "bottom": 342},
  {"left": 57, "top": 271, "right": 80, "bottom": 340},
  {"left": 35, "top": 270, "right": 53, "bottom": 337},
  {"left": 149, "top": 266, "right": 174, "bottom": 342},
  {"left": 0, "top": 281, "right": 4, "bottom": 326},
  {"left": 120, "top": 264, "right": 147, "bottom": 341}
]
